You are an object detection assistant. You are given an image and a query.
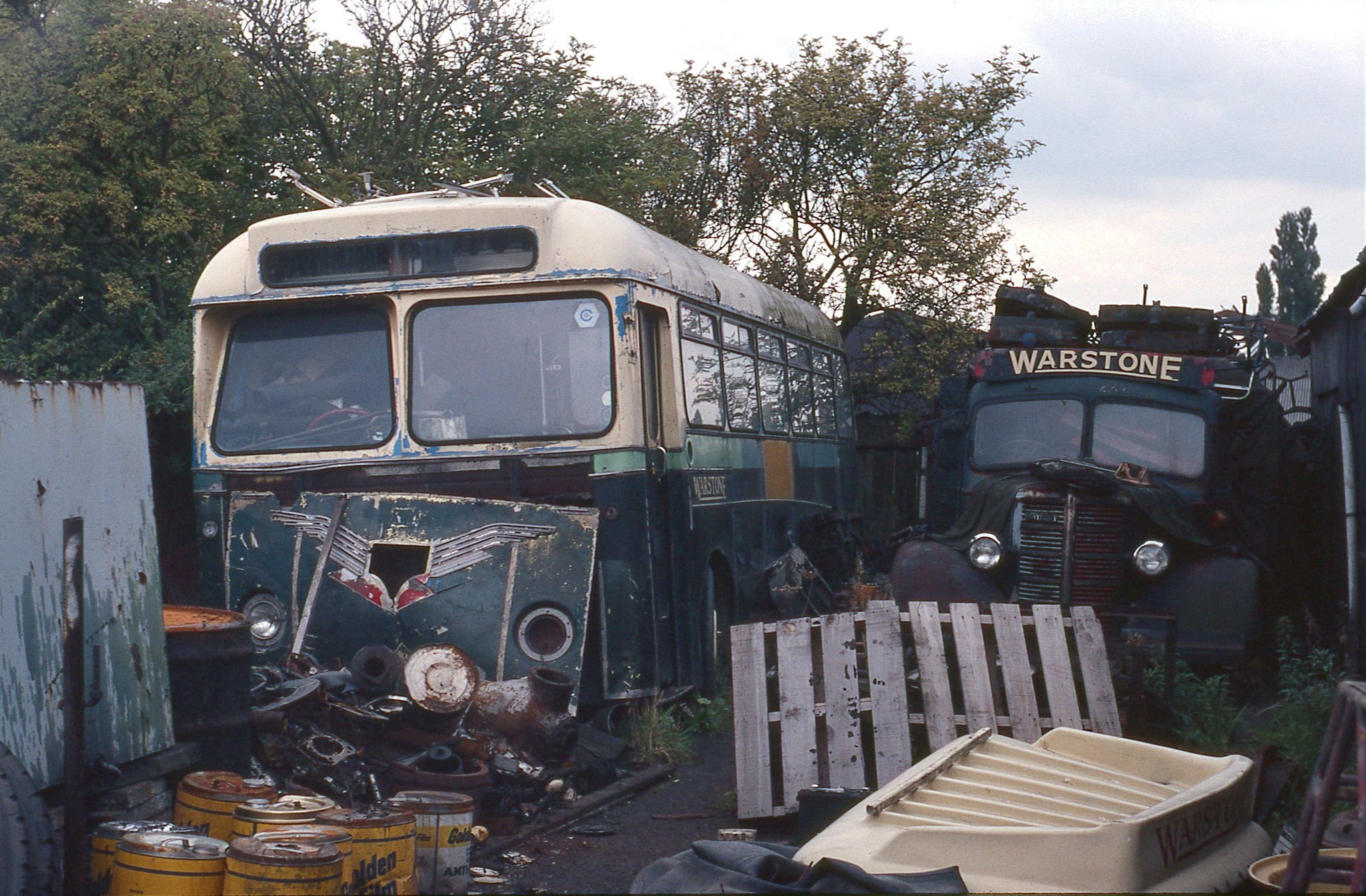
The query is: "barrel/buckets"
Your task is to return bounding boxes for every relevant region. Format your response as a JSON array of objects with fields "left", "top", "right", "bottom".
[
  {"left": 165, "top": 608, "right": 252, "bottom": 770},
  {"left": 88, "top": 772, "right": 474, "bottom": 896}
]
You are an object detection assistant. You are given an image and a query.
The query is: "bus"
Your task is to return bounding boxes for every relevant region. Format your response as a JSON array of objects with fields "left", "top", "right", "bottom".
[
  {"left": 186, "top": 167, "right": 860, "bottom": 772},
  {"left": 884, "top": 278, "right": 1289, "bottom": 697}
]
